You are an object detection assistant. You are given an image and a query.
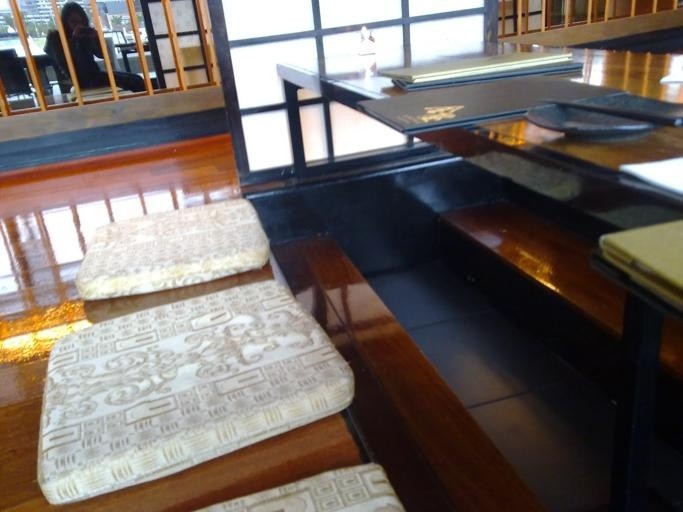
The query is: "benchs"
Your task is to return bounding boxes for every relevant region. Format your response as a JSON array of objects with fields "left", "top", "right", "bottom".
[{"left": 295, "top": 226, "right": 552, "bottom": 511}]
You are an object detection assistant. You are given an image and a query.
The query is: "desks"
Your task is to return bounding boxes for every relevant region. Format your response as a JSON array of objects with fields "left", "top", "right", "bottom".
[{"left": 276, "top": 33, "right": 683, "bottom": 460}]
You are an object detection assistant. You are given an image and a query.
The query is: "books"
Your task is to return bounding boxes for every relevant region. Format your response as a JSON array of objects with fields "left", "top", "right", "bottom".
[
  {"left": 600, "top": 221, "right": 683, "bottom": 309},
  {"left": 354, "top": 76, "right": 630, "bottom": 136},
  {"left": 375, "top": 52, "right": 573, "bottom": 82},
  {"left": 375, "top": 62, "right": 585, "bottom": 91}
]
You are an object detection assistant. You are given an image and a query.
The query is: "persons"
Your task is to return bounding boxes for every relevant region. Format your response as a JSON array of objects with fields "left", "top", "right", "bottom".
[{"left": 43, "top": 2, "right": 146, "bottom": 92}]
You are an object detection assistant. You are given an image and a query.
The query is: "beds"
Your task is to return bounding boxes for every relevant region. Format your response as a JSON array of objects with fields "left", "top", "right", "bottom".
[{"left": 0, "top": 131, "right": 399, "bottom": 512}]
